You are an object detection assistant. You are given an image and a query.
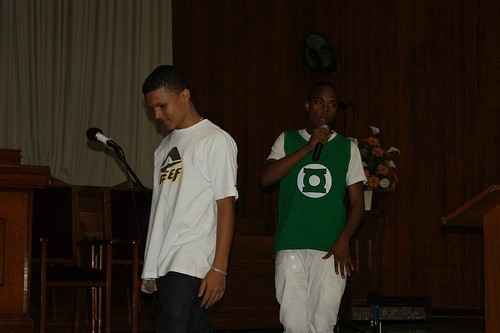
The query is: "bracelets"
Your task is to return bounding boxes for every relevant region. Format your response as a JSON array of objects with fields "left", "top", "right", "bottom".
[{"left": 259, "top": 81, "right": 369, "bottom": 333}]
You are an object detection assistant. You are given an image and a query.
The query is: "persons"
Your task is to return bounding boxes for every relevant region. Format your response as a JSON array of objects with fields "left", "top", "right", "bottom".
[{"left": 129, "top": 65, "right": 239, "bottom": 333}]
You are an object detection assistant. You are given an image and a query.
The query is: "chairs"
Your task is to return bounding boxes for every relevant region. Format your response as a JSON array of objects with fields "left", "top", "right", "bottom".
[{"left": 40, "top": 236, "right": 116, "bottom": 332}]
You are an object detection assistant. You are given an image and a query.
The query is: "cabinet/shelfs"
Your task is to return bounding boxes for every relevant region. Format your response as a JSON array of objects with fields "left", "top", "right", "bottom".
[{"left": 0, "top": 147, "right": 152, "bottom": 332}]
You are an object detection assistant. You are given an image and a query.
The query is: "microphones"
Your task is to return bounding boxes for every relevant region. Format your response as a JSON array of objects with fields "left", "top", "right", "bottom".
[
  {"left": 145, "top": 282, "right": 153, "bottom": 292},
  {"left": 313, "top": 125, "right": 330, "bottom": 163},
  {"left": 86, "top": 128, "right": 122, "bottom": 150}
]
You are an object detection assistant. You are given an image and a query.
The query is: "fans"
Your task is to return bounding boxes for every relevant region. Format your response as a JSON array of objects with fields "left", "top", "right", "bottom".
[{"left": 301, "top": 32, "right": 341, "bottom": 74}]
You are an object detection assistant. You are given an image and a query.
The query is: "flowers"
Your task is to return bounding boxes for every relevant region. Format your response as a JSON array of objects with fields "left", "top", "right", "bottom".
[{"left": 355, "top": 137, "right": 398, "bottom": 193}]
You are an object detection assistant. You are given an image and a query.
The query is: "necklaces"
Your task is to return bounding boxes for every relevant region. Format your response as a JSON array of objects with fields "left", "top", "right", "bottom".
[{"left": 211, "top": 265, "right": 228, "bottom": 275}]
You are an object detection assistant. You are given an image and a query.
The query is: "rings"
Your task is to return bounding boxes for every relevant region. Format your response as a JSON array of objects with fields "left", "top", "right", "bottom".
[{"left": 318, "top": 131, "right": 322, "bottom": 135}]
